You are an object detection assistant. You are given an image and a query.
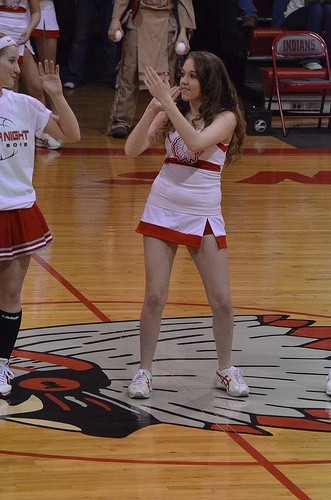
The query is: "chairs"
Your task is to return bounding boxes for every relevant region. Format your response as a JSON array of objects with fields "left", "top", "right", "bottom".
[{"left": 262, "top": 31, "right": 331, "bottom": 137}]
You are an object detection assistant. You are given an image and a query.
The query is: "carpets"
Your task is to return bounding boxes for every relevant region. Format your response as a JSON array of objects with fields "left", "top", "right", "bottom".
[{"left": 271, "top": 127, "right": 331, "bottom": 149}]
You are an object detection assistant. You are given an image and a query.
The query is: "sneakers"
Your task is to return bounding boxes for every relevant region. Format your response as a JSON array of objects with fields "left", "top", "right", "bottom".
[
  {"left": 213, "top": 365, "right": 249, "bottom": 397},
  {"left": 0, "top": 357, "right": 14, "bottom": 397},
  {"left": 304, "top": 62, "right": 322, "bottom": 70},
  {"left": 111, "top": 127, "right": 128, "bottom": 137},
  {"left": 62, "top": 81, "right": 74, "bottom": 88},
  {"left": 35, "top": 134, "right": 61, "bottom": 149},
  {"left": 127, "top": 369, "right": 153, "bottom": 399},
  {"left": 324, "top": 372, "right": 331, "bottom": 395}
]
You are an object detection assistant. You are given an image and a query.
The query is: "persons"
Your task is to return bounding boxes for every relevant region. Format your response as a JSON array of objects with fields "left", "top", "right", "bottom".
[
  {"left": 283, "top": 0, "right": 331, "bottom": 70},
  {"left": 238, "top": 0, "right": 290, "bottom": 28},
  {"left": 106, "top": 0, "right": 196, "bottom": 137},
  {"left": 125, "top": 51, "right": 249, "bottom": 399},
  {"left": 0, "top": 0, "right": 62, "bottom": 149},
  {"left": 0, "top": 33, "right": 80, "bottom": 397},
  {"left": 62, "top": 0, "right": 120, "bottom": 89},
  {"left": 31, "top": 0, "right": 60, "bottom": 115}
]
